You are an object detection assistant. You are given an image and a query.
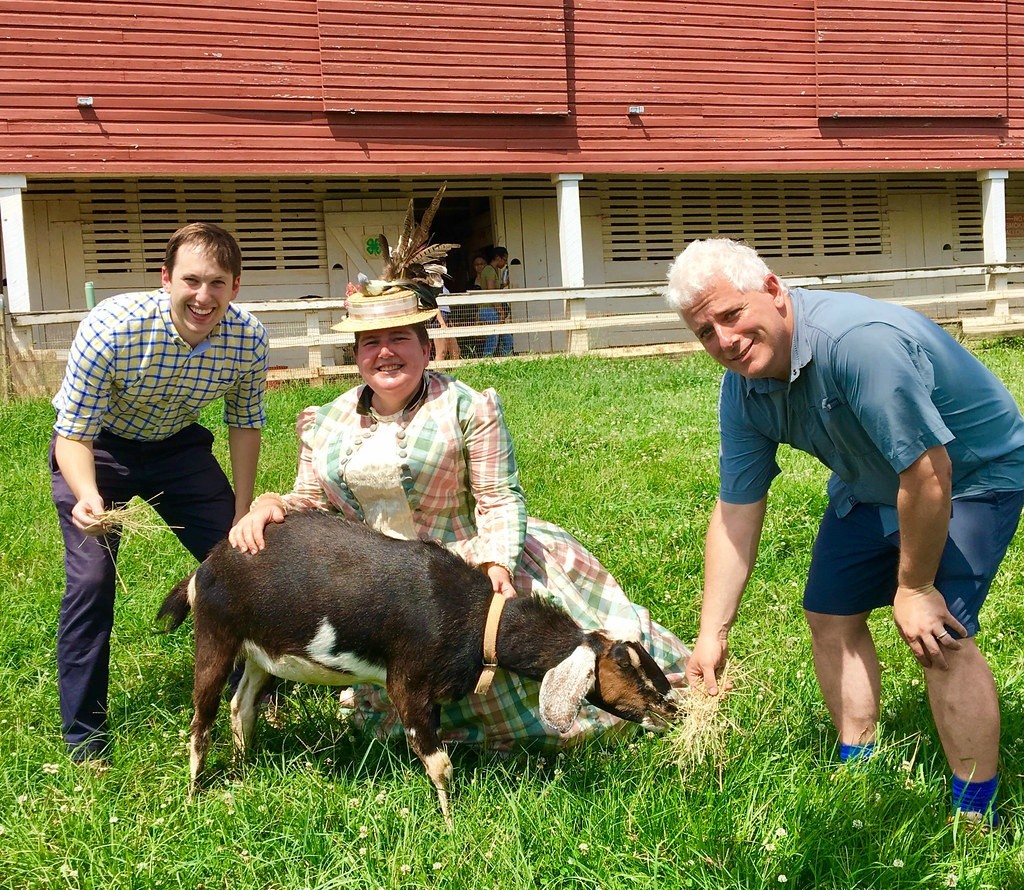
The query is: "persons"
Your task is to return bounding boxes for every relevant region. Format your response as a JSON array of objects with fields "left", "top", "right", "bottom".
[
  {"left": 424, "top": 246, "right": 510, "bottom": 361},
  {"left": 47, "top": 223, "right": 270, "bottom": 782},
  {"left": 666, "top": 239, "right": 1024, "bottom": 850},
  {"left": 228, "top": 276, "right": 698, "bottom": 740}
]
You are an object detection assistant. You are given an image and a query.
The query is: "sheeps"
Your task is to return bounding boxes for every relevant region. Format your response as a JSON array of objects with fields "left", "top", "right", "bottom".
[{"left": 154, "top": 511, "right": 680, "bottom": 833}]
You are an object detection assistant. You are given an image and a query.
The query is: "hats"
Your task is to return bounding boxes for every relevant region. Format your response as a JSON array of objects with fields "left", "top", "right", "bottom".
[{"left": 328, "top": 179, "right": 459, "bottom": 331}]
